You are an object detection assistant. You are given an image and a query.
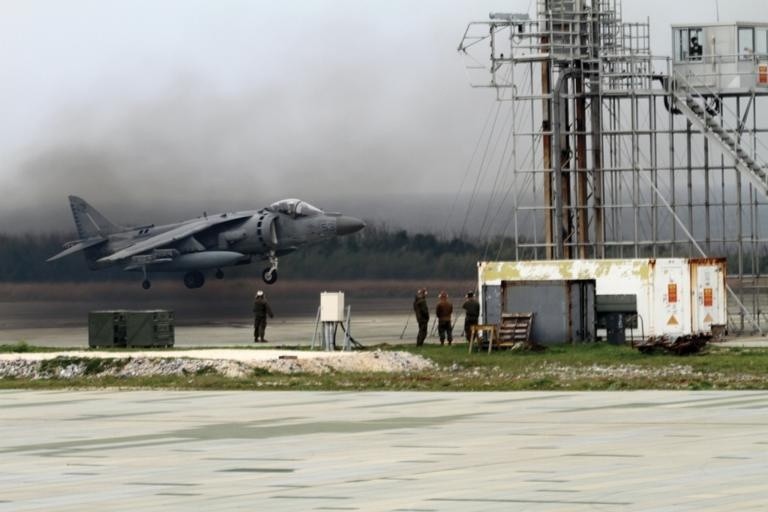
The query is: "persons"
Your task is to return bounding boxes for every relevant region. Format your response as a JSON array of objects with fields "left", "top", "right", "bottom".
[
  {"left": 253, "top": 290, "right": 274, "bottom": 342},
  {"left": 414, "top": 288, "right": 429, "bottom": 346},
  {"left": 436, "top": 292, "right": 453, "bottom": 346},
  {"left": 689, "top": 37, "right": 701, "bottom": 60},
  {"left": 462, "top": 291, "right": 480, "bottom": 343}
]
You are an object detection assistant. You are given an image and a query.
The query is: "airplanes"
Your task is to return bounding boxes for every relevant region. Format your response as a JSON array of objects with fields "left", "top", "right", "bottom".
[{"left": 43, "top": 191, "right": 369, "bottom": 295}]
